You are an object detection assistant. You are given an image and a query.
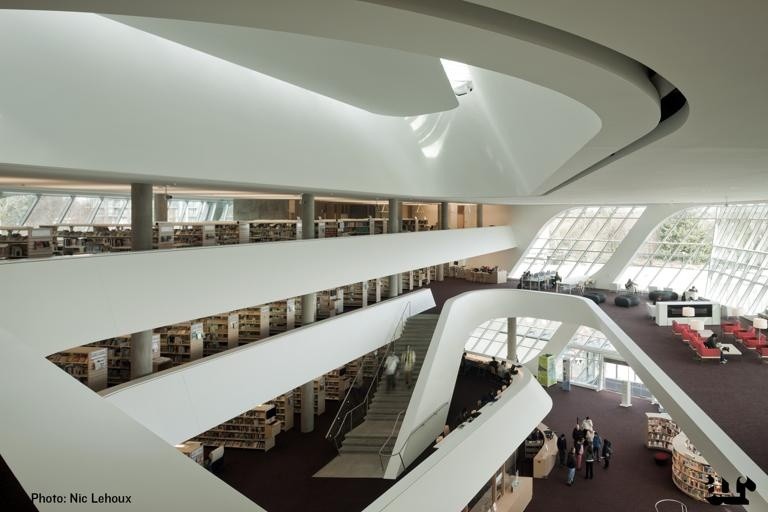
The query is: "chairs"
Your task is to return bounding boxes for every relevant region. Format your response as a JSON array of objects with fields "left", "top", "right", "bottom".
[
  {"left": 576, "top": 278, "right": 594, "bottom": 297},
  {"left": 671, "top": 314, "right": 768, "bottom": 366},
  {"left": 451, "top": 266, "right": 490, "bottom": 284},
  {"left": 685, "top": 291, "right": 699, "bottom": 301},
  {"left": 625, "top": 284, "right": 637, "bottom": 295},
  {"left": 647, "top": 302, "right": 656, "bottom": 321},
  {"left": 436, "top": 360, "right": 512, "bottom": 445}
]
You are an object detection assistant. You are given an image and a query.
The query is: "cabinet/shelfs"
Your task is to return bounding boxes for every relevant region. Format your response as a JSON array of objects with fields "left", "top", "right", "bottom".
[
  {"left": 644, "top": 412, "right": 733, "bottom": 506},
  {"left": 0, "top": 217, "right": 445, "bottom": 470}
]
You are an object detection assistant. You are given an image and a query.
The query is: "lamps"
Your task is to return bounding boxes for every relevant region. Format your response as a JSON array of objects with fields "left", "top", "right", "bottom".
[{"left": 681, "top": 307, "right": 695, "bottom": 326}]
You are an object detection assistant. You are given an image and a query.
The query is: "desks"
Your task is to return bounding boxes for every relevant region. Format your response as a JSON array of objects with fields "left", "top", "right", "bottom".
[
  {"left": 488, "top": 268, "right": 507, "bottom": 284},
  {"left": 521, "top": 273, "right": 556, "bottom": 290},
  {"left": 556, "top": 280, "right": 579, "bottom": 295},
  {"left": 649, "top": 291, "right": 679, "bottom": 301}
]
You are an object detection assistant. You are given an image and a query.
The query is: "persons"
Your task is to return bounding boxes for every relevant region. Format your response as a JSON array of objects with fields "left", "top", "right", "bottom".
[
  {"left": 551, "top": 271, "right": 561, "bottom": 288},
  {"left": 436, "top": 356, "right": 516, "bottom": 442},
  {"left": 584, "top": 445, "right": 594, "bottom": 479},
  {"left": 601, "top": 438, "right": 612, "bottom": 468},
  {"left": 525, "top": 271, "right": 532, "bottom": 277},
  {"left": 400, "top": 344, "right": 417, "bottom": 389},
  {"left": 575, "top": 437, "right": 586, "bottom": 471},
  {"left": 626, "top": 278, "right": 638, "bottom": 293},
  {"left": 704, "top": 332, "right": 727, "bottom": 364},
  {"left": 572, "top": 414, "right": 602, "bottom": 463},
  {"left": 384, "top": 350, "right": 401, "bottom": 393},
  {"left": 462, "top": 352, "right": 467, "bottom": 362},
  {"left": 565, "top": 447, "right": 577, "bottom": 486},
  {"left": 556, "top": 433, "right": 567, "bottom": 470}
]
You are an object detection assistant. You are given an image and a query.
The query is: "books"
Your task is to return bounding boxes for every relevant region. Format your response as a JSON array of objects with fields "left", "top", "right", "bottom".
[{"left": 715, "top": 341, "right": 724, "bottom": 349}]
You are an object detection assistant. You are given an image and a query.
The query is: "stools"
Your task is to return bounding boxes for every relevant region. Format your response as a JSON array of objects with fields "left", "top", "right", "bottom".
[
  {"left": 584, "top": 292, "right": 606, "bottom": 304},
  {"left": 615, "top": 294, "right": 641, "bottom": 308}
]
